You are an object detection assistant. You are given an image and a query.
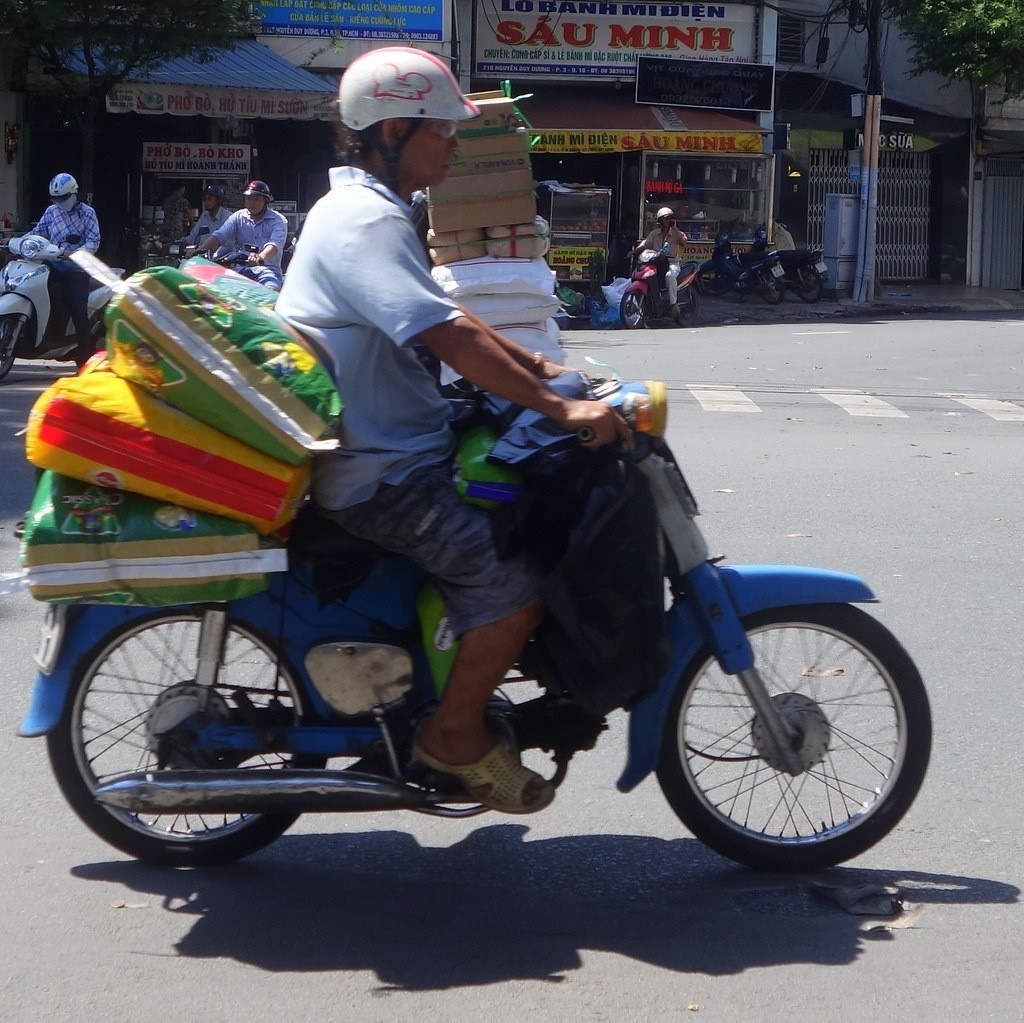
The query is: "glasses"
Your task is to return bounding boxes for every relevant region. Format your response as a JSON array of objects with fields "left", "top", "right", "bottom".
[
  {"left": 397, "top": 114, "right": 460, "bottom": 139},
  {"left": 50, "top": 192, "right": 72, "bottom": 202}
]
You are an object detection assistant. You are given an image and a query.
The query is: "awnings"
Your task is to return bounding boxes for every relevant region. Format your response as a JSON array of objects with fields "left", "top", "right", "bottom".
[{"left": 18, "top": 39, "right": 343, "bottom": 121}]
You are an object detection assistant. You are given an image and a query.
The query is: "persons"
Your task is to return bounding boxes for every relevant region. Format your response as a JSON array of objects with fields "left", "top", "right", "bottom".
[
  {"left": 627, "top": 207, "right": 688, "bottom": 316},
  {"left": 175, "top": 184, "right": 235, "bottom": 262},
  {"left": 274, "top": 46, "right": 635, "bottom": 813},
  {"left": 185, "top": 180, "right": 287, "bottom": 293},
  {"left": 22, "top": 173, "right": 101, "bottom": 370},
  {"left": 286, "top": 186, "right": 435, "bottom": 271},
  {"left": 161, "top": 181, "right": 195, "bottom": 242}
]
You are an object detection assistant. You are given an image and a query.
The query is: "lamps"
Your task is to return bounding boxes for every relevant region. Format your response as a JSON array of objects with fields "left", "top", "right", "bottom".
[{"left": 651, "top": 158, "right": 758, "bottom": 185}]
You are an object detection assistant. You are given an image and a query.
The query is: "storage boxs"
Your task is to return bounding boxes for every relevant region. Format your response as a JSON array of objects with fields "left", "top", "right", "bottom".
[
  {"left": 458, "top": 97, "right": 518, "bottom": 136},
  {"left": 424, "top": 169, "right": 537, "bottom": 229},
  {"left": 447, "top": 130, "right": 530, "bottom": 174},
  {"left": 464, "top": 90, "right": 505, "bottom": 101}
]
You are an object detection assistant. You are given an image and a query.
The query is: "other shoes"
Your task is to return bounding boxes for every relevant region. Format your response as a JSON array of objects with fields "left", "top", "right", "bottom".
[{"left": 672, "top": 303, "right": 681, "bottom": 316}]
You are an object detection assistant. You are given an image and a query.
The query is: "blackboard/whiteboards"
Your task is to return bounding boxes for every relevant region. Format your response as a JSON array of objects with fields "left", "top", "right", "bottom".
[{"left": 635, "top": 55, "right": 775, "bottom": 113}]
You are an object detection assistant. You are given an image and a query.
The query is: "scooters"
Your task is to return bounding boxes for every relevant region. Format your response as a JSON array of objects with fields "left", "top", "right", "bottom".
[
  {"left": 618, "top": 242, "right": 705, "bottom": 329},
  {"left": 157, "top": 225, "right": 267, "bottom": 284},
  {"left": 699, "top": 216, "right": 786, "bottom": 305},
  {"left": 1, "top": 232, "right": 126, "bottom": 384}
]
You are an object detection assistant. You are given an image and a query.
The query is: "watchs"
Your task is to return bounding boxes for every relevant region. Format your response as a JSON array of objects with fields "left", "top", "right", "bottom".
[{"left": 532, "top": 352, "right": 544, "bottom": 376}]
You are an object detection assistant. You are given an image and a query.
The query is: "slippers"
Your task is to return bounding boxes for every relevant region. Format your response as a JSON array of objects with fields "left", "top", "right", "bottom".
[{"left": 412, "top": 738, "right": 555, "bottom": 815}]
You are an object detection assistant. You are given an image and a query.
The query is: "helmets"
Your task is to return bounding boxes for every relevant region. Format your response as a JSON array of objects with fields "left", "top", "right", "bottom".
[
  {"left": 657, "top": 207, "right": 673, "bottom": 221},
  {"left": 338, "top": 46, "right": 482, "bottom": 130},
  {"left": 49, "top": 173, "right": 79, "bottom": 196},
  {"left": 202, "top": 184, "right": 223, "bottom": 201},
  {"left": 243, "top": 180, "right": 271, "bottom": 203}
]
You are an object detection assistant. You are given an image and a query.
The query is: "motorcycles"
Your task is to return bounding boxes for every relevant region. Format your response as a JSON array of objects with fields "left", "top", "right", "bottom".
[
  {"left": 749, "top": 220, "right": 831, "bottom": 305},
  {"left": 13, "top": 372, "right": 935, "bottom": 877}
]
